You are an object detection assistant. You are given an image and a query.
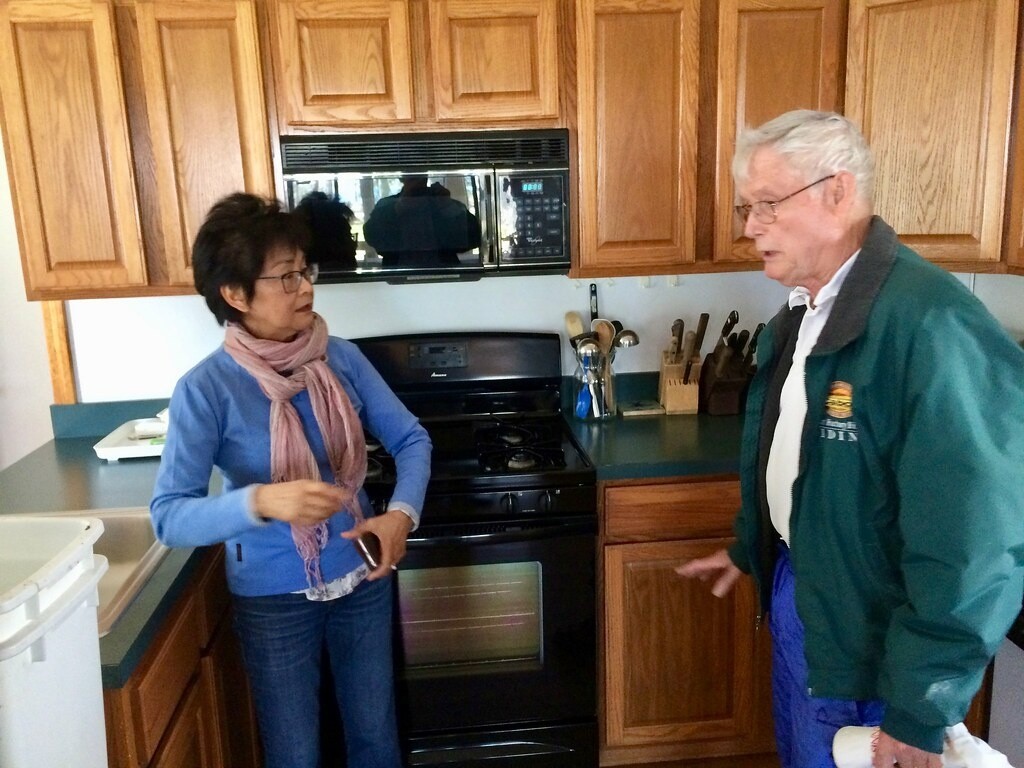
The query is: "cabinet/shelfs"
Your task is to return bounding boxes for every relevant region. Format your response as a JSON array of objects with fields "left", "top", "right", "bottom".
[
  {"left": 102, "top": 546, "right": 264, "bottom": 768},
  {"left": 0, "top": 0, "right": 1024, "bottom": 303},
  {"left": 596, "top": 475, "right": 776, "bottom": 768}
]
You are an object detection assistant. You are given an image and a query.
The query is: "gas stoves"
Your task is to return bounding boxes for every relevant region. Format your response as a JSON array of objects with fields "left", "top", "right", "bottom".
[{"left": 347, "top": 332, "right": 596, "bottom": 487}]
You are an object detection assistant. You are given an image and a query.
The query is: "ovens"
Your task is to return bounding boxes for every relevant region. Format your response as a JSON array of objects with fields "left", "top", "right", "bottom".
[{"left": 392, "top": 518, "right": 599, "bottom": 768}]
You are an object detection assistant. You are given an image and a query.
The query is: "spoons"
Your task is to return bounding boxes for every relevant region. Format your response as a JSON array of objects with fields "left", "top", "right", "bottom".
[{"left": 564, "top": 312, "right": 640, "bottom": 419}]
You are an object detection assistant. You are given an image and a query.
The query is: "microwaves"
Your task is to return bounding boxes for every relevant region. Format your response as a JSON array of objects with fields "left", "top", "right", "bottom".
[{"left": 281, "top": 127, "right": 572, "bottom": 285}]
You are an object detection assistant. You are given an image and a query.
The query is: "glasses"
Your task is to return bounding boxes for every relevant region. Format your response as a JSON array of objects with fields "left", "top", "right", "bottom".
[
  {"left": 249, "top": 260, "right": 319, "bottom": 294},
  {"left": 733, "top": 175, "right": 835, "bottom": 227}
]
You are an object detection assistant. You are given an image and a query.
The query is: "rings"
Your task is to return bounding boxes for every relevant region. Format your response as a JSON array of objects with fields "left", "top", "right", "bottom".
[{"left": 389, "top": 562, "right": 397, "bottom": 570}]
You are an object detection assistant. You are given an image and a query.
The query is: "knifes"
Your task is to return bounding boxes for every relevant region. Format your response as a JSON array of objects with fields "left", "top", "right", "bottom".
[{"left": 667, "top": 310, "right": 766, "bottom": 385}]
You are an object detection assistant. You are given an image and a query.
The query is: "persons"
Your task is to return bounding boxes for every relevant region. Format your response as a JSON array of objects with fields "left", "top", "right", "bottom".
[
  {"left": 149, "top": 189, "right": 434, "bottom": 768},
  {"left": 363, "top": 174, "right": 482, "bottom": 268},
  {"left": 294, "top": 191, "right": 358, "bottom": 272},
  {"left": 675, "top": 108, "right": 1024, "bottom": 768}
]
